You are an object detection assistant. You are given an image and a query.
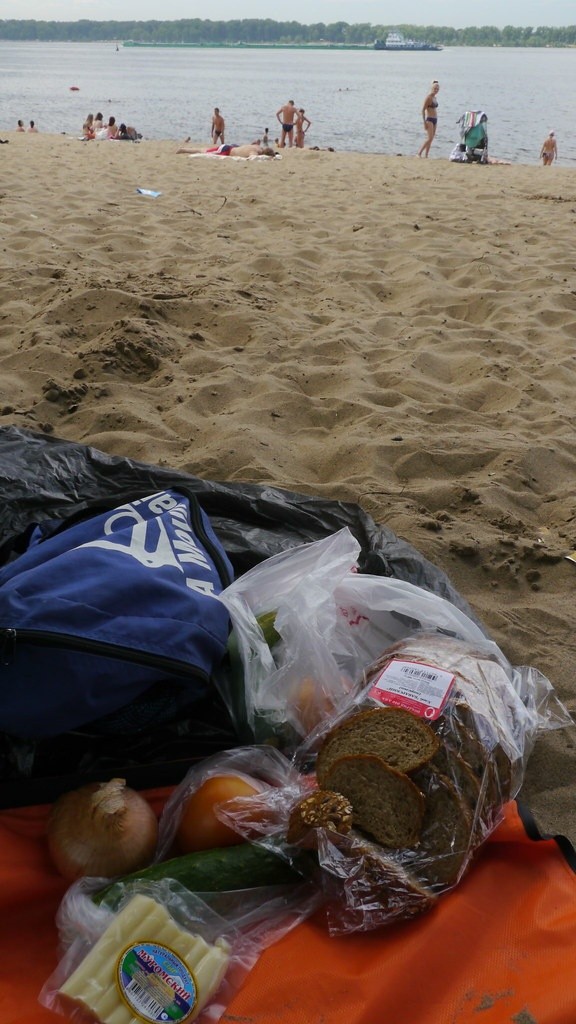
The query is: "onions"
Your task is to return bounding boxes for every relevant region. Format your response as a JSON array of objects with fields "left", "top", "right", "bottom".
[{"left": 45, "top": 776, "right": 155, "bottom": 884}]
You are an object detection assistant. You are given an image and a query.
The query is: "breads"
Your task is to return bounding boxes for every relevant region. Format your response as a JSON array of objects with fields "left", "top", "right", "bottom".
[{"left": 287, "top": 635, "right": 530, "bottom": 909}]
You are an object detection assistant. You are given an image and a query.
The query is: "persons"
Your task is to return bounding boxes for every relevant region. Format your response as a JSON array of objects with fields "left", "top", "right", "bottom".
[
  {"left": 27, "top": 120, "right": 38, "bottom": 132},
  {"left": 82, "top": 112, "right": 127, "bottom": 137},
  {"left": 251, "top": 128, "right": 269, "bottom": 146},
  {"left": 211, "top": 108, "right": 225, "bottom": 144},
  {"left": 184, "top": 136, "right": 191, "bottom": 144},
  {"left": 16, "top": 120, "right": 25, "bottom": 132},
  {"left": 274, "top": 100, "right": 311, "bottom": 148},
  {"left": 540, "top": 131, "right": 557, "bottom": 166},
  {"left": 418, "top": 80, "right": 440, "bottom": 158},
  {"left": 176, "top": 144, "right": 275, "bottom": 158}
]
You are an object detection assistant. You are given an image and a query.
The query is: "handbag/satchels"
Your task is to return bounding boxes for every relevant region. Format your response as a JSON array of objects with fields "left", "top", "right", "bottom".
[{"left": 0, "top": 484, "right": 238, "bottom": 744}]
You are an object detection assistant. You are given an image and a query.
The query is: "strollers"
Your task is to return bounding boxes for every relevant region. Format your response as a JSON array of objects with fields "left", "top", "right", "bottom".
[{"left": 462, "top": 111, "right": 489, "bottom": 164}]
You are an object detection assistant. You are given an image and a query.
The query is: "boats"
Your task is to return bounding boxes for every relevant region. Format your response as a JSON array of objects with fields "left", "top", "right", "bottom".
[{"left": 375, "top": 32, "right": 441, "bottom": 50}]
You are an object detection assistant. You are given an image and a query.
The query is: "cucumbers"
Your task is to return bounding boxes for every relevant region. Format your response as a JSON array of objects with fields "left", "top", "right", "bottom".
[{"left": 88, "top": 832, "right": 300, "bottom": 911}]
